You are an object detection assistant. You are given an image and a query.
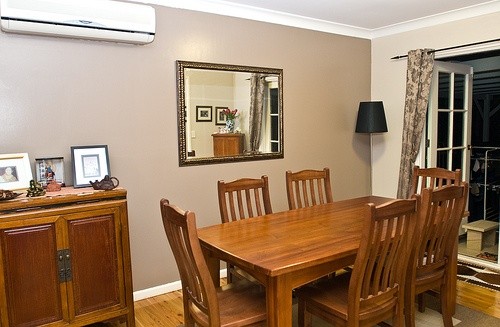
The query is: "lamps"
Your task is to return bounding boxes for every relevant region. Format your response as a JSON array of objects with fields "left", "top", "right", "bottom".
[{"left": 355, "top": 101, "right": 388, "bottom": 196}]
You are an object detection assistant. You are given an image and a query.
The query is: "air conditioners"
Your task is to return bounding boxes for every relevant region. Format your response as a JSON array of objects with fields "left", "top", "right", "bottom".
[{"left": 0, "top": 0, "right": 157, "bottom": 47}]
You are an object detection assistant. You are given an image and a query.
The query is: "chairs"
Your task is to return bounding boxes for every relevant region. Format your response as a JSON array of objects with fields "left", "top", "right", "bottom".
[{"left": 160, "top": 166, "right": 468, "bottom": 327}]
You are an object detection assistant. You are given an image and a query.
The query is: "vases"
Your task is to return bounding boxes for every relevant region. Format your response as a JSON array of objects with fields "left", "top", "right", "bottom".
[{"left": 226, "top": 118, "right": 235, "bottom": 134}]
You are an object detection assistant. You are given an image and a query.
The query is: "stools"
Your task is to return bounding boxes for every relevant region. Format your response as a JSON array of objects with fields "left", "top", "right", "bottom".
[{"left": 462, "top": 220, "right": 499, "bottom": 251}]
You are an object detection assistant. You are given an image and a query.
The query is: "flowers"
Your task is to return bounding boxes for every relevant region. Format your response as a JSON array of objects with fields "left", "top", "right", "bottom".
[{"left": 223, "top": 109, "right": 240, "bottom": 119}]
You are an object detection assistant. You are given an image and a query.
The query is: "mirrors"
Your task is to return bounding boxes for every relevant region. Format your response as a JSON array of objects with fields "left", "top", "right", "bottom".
[{"left": 176, "top": 60, "right": 284, "bottom": 167}]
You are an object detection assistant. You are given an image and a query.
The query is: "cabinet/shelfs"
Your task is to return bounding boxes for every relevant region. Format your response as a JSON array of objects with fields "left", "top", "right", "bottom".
[{"left": 0, "top": 185, "right": 135, "bottom": 327}]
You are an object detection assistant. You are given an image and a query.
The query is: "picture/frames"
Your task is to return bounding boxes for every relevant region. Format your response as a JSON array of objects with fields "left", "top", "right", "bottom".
[
  {"left": 70, "top": 145, "right": 111, "bottom": 189},
  {"left": 196, "top": 106, "right": 212, "bottom": 122},
  {"left": 0, "top": 153, "right": 34, "bottom": 191},
  {"left": 215, "top": 107, "right": 228, "bottom": 125},
  {"left": 35, "top": 157, "right": 65, "bottom": 187}
]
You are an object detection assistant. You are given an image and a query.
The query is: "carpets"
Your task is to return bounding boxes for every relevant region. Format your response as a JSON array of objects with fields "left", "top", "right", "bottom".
[{"left": 293, "top": 290, "right": 500, "bottom": 327}]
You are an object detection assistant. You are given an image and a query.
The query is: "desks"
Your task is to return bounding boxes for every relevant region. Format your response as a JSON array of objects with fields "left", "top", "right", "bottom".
[{"left": 197, "top": 196, "right": 470, "bottom": 327}]
